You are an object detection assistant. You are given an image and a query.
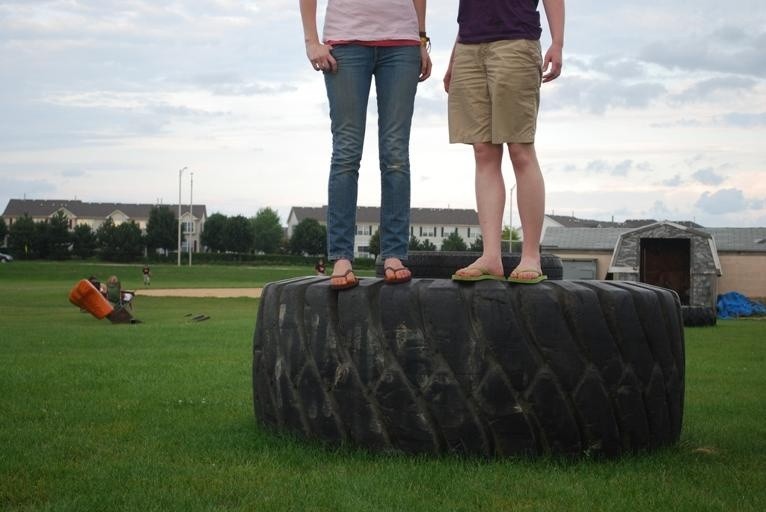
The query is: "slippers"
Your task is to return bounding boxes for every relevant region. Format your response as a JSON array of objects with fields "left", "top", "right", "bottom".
[
  {"left": 451, "top": 267, "right": 547, "bottom": 284},
  {"left": 330, "top": 269, "right": 359, "bottom": 290},
  {"left": 383, "top": 267, "right": 412, "bottom": 283}
]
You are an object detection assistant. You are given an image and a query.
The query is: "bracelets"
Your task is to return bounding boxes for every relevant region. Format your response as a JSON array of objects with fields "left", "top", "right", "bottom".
[{"left": 419, "top": 32, "right": 428, "bottom": 38}]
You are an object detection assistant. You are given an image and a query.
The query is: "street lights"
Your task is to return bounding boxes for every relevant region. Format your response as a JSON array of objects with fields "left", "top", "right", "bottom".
[
  {"left": 178, "top": 167, "right": 193, "bottom": 267},
  {"left": 510, "top": 183, "right": 516, "bottom": 252}
]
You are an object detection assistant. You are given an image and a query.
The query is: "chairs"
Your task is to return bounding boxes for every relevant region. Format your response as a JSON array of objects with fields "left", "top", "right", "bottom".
[{"left": 90, "top": 280, "right": 121, "bottom": 305}]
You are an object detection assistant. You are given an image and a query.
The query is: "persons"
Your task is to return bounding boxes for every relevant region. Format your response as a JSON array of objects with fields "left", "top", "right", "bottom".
[
  {"left": 143, "top": 264, "right": 151, "bottom": 285},
  {"left": 299, "top": 0, "right": 432, "bottom": 290},
  {"left": 89, "top": 275, "right": 134, "bottom": 302},
  {"left": 443, "top": 0, "right": 566, "bottom": 285},
  {"left": 315, "top": 258, "right": 325, "bottom": 276}
]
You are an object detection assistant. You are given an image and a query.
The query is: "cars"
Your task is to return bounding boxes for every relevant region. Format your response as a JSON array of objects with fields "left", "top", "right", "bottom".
[{"left": 0, "top": 253, "right": 13, "bottom": 264}]
